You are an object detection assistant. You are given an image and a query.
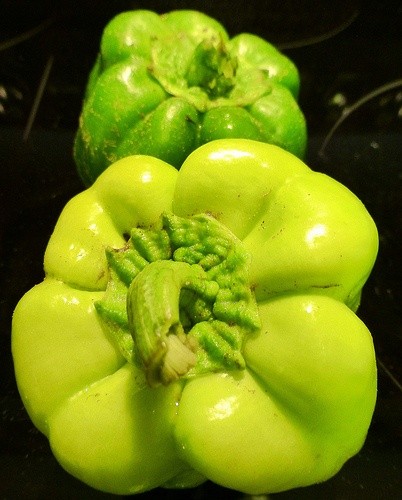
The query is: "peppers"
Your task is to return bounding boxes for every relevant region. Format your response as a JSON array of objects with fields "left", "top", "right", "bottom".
[
  {"left": 72, "top": 7, "right": 308, "bottom": 191},
  {"left": 6, "top": 141, "right": 382, "bottom": 498}
]
